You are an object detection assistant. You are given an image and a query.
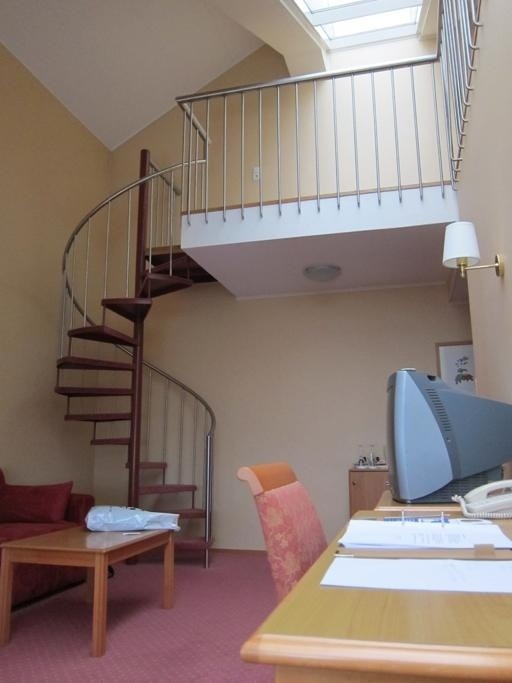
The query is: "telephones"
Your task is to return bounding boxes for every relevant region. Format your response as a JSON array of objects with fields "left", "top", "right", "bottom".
[{"left": 451, "top": 479, "right": 512, "bottom": 519}]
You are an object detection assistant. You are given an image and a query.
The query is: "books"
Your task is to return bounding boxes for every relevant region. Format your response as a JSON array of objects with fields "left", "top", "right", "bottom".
[{"left": 337, "top": 516, "right": 511, "bottom": 549}]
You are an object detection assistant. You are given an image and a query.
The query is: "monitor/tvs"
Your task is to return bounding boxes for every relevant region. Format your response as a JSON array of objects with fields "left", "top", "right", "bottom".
[{"left": 386, "top": 368, "right": 512, "bottom": 505}]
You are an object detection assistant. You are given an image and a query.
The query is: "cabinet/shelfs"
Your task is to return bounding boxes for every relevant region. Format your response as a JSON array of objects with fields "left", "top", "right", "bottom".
[{"left": 348, "top": 470, "right": 392, "bottom": 515}]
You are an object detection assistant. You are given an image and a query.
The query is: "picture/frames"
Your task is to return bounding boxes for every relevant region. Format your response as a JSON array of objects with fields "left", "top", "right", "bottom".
[{"left": 434, "top": 340, "right": 474, "bottom": 384}]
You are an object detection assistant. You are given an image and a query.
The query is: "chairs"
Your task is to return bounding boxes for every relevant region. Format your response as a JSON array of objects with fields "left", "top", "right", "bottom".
[
  {"left": 0, "top": 468, "right": 94, "bottom": 608},
  {"left": 237, "top": 461, "right": 328, "bottom": 600}
]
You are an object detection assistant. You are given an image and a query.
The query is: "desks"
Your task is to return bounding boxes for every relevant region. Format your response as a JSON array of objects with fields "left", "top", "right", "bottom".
[
  {"left": 376, "top": 487, "right": 470, "bottom": 509},
  {"left": 239, "top": 512, "right": 512, "bottom": 683},
  {"left": 0, "top": 528, "right": 175, "bottom": 656}
]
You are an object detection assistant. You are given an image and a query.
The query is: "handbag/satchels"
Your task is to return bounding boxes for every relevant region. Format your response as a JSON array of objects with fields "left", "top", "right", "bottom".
[{"left": 85, "top": 506, "right": 181, "bottom": 531}]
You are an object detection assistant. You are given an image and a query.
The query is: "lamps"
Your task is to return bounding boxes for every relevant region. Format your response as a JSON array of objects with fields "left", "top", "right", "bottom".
[
  {"left": 442, "top": 221, "right": 505, "bottom": 278},
  {"left": 304, "top": 265, "right": 341, "bottom": 282}
]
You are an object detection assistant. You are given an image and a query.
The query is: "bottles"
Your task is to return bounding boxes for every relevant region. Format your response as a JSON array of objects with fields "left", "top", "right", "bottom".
[{"left": 354, "top": 441, "right": 382, "bottom": 470}]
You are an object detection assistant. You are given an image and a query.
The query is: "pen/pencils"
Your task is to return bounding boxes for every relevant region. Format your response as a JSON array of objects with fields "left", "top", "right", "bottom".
[{"left": 384, "top": 516, "right": 449, "bottom": 523}]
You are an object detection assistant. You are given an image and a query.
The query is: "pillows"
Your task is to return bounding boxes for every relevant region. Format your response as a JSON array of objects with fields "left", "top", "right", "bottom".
[{"left": 0, "top": 480, "right": 73, "bottom": 524}]
